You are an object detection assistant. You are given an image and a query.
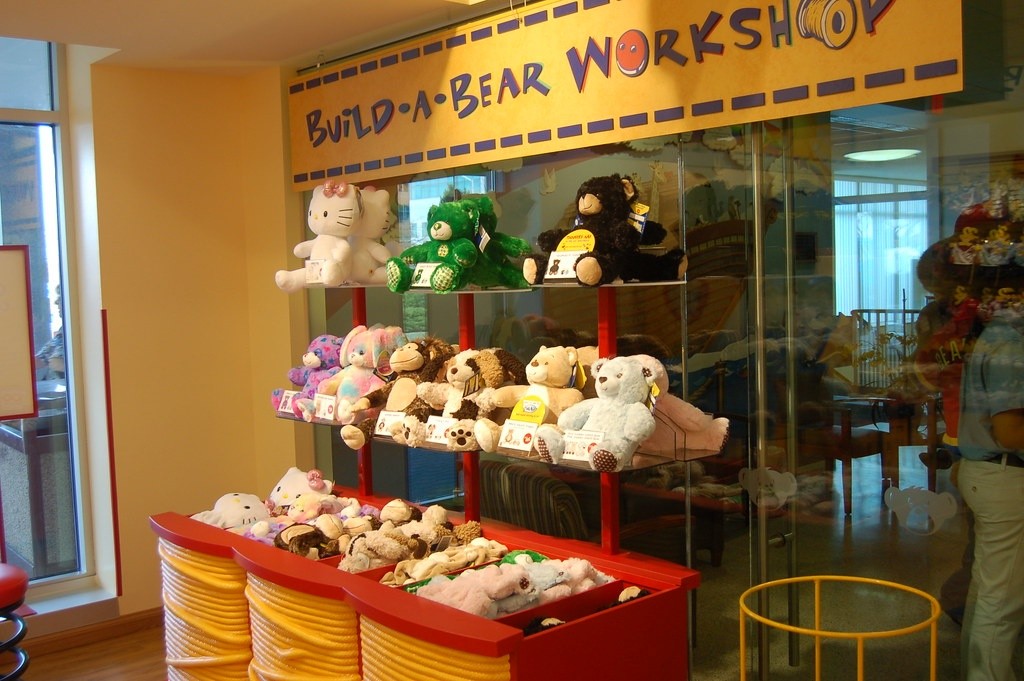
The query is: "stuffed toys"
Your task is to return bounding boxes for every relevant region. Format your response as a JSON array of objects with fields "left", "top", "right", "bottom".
[
  {"left": 273, "top": 183, "right": 364, "bottom": 290},
  {"left": 273, "top": 324, "right": 656, "bottom": 469},
  {"left": 907, "top": 204, "right": 1024, "bottom": 443},
  {"left": 384, "top": 200, "right": 480, "bottom": 293},
  {"left": 520, "top": 173, "right": 636, "bottom": 286},
  {"left": 646, "top": 452, "right": 850, "bottom": 513},
  {"left": 349, "top": 186, "right": 396, "bottom": 283},
  {"left": 189, "top": 466, "right": 652, "bottom": 637},
  {"left": 613, "top": 209, "right": 688, "bottom": 281},
  {"left": 463, "top": 195, "right": 530, "bottom": 289},
  {"left": 370, "top": 325, "right": 731, "bottom": 452}
]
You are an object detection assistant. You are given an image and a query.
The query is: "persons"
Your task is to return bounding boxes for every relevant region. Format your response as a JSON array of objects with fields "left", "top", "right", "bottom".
[{"left": 957, "top": 278, "right": 1023, "bottom": 681}]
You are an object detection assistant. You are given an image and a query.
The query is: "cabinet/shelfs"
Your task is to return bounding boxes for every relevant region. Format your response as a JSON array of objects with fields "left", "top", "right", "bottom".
[{"left": 150, "top": 133, "right": 704, "bottom": 681}]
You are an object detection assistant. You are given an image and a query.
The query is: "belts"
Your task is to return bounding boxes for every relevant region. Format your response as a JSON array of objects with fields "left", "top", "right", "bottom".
[{"left": 988, "top": 454, "right": 1024, "bottom": 469}]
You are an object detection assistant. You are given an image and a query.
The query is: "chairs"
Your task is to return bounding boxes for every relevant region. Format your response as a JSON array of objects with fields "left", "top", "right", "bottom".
[
  {"left": 478, "top": 461, "right": 697, "bottom": 647},
  {"left": 764, "top": 371, "right": 896, "bottom": 516}
]
